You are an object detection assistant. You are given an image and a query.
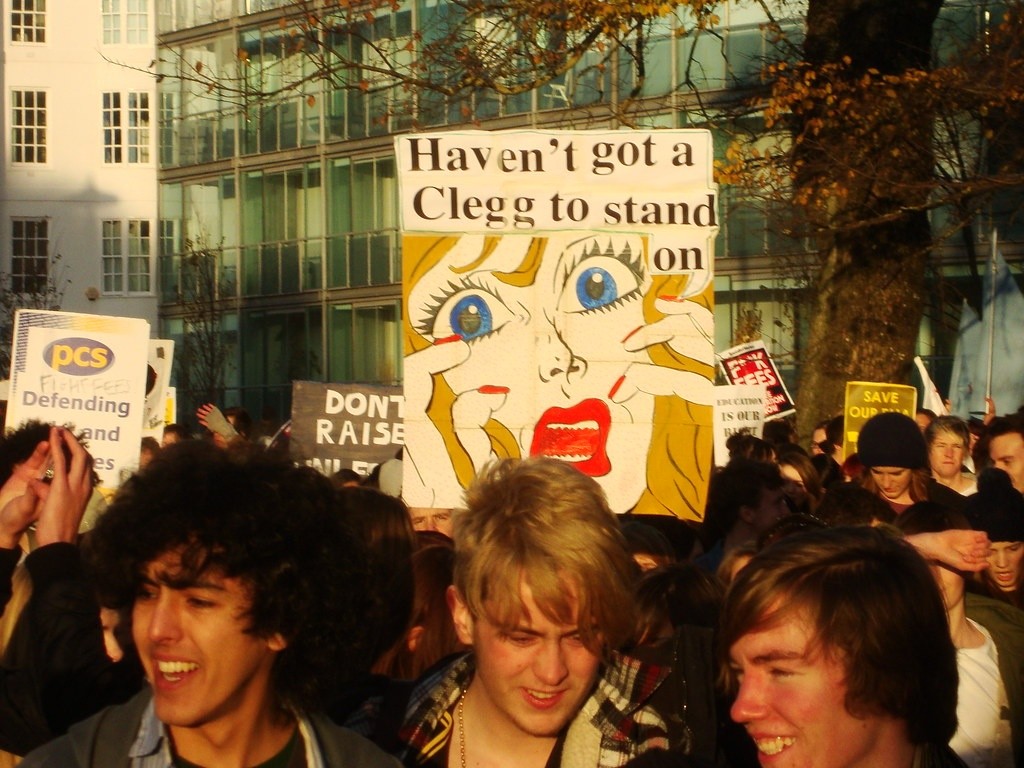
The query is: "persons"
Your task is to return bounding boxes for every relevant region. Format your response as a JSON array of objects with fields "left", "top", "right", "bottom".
[{"left": 0, "top": 403, "right": 1024, "bottom": 768}]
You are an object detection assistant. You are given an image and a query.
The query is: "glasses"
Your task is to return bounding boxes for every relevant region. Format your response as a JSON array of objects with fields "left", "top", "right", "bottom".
[{"left": 809, "top": 438, "right": 818, "bottom": 448}]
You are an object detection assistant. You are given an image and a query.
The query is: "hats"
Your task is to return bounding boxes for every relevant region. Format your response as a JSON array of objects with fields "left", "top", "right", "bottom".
[
  {"left": 857, "top": 411, "right": 927, "bottom": 470},
  {"left": 959, "top": 466, "right": 1024, "bottom": 543}
]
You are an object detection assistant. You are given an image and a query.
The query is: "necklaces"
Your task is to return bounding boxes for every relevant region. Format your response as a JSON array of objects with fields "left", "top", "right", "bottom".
[{"left": 459, "top": 689, "right": 466, "bottom": 768}]
